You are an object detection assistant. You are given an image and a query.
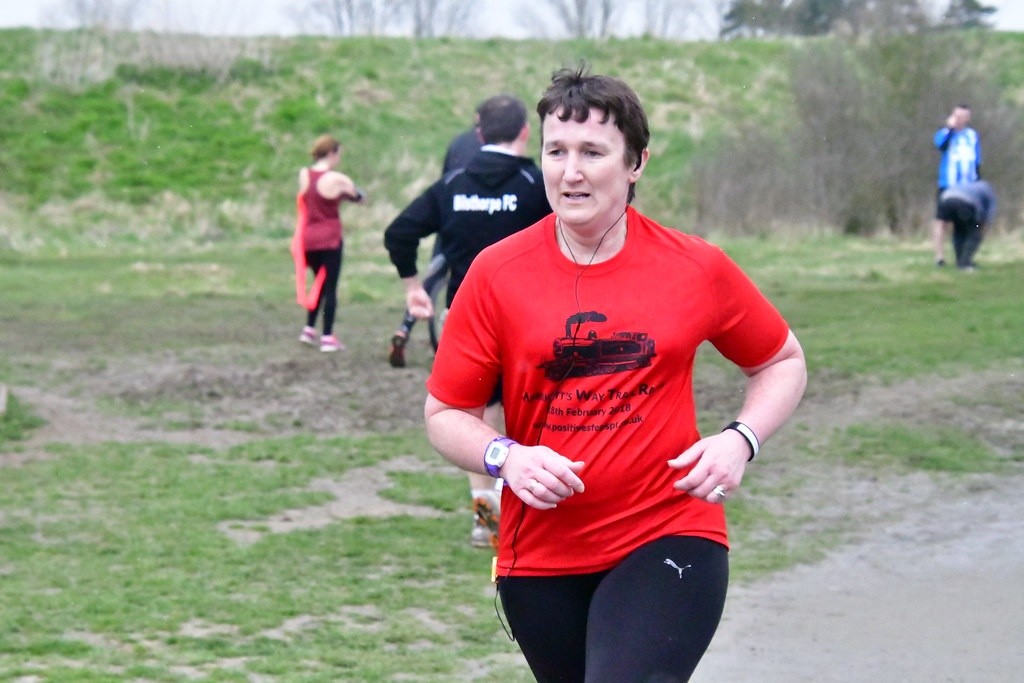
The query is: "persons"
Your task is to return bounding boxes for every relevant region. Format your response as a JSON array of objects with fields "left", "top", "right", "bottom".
[
  {"left": 422, "top": 58, "right": 811, "bottom": 683},
  {"left": 931, "top": 103, "right": 983, "bottom": 267},
  {"left": 934, "top": 180, "right": 997, "bottom": 270},
  {"left": 290, "top": 133, "right": 367, "bottom": 354},
  {"left": 383, "top": 97, "right": 562, "bottom": 546},
  {"left": 385, "top": 97, "right": 517, "bottom": 369}
]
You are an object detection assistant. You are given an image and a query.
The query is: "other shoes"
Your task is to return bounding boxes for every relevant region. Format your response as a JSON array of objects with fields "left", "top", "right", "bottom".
[{"left": 936, "top": 260, "right": 946, "bottom": 267}]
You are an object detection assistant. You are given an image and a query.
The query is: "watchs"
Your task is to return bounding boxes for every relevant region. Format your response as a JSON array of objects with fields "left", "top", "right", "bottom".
[{"left": 483, "top": 434, "right": 517, "bottom": 478}]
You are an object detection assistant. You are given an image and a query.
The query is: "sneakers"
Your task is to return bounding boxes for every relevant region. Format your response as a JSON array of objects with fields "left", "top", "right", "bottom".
[
  {"left": 320, "top": 334, "right": 347, "bottom": 352},
  {"left": 475, "top": 493, "right": 505, "bottom": 548},
  {"left": 389, "top": 330, "right": 407, "bottom": 368},
  {"left": 299, "top": 325, "right": 316, "bottom": 346},
  {"left": 471, "top": 515, "right": 492, "bottom": 546}
]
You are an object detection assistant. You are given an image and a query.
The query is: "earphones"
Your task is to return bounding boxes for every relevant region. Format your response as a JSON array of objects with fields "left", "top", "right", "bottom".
[{"left": 634, "top": 154, "right": 641, "bottom": 171}]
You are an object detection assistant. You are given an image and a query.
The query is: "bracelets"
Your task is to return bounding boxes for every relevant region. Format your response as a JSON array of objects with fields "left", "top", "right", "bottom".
[{"left": 724, "top": 419, "right": 760, "bottom": 463}]
